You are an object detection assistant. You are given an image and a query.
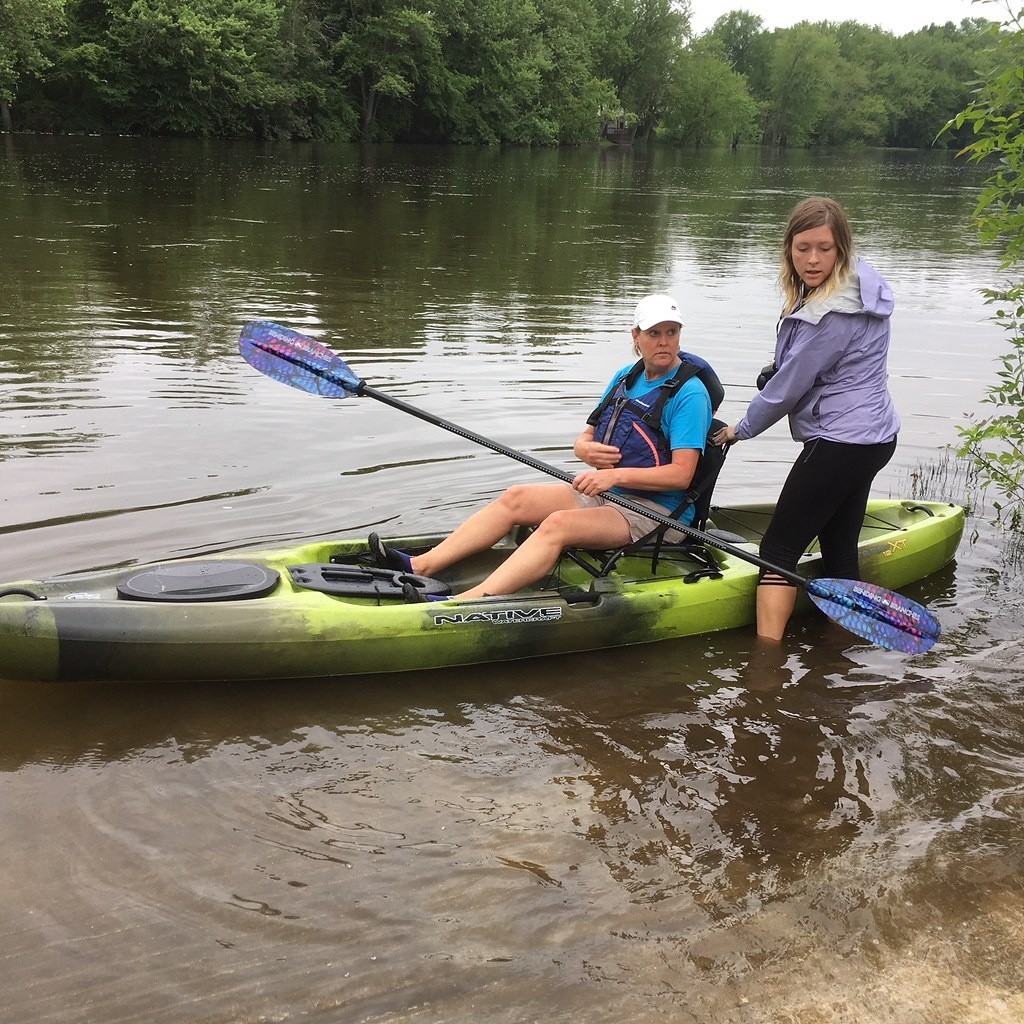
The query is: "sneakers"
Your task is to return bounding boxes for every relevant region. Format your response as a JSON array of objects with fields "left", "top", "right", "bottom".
[
  {"left": 402, "top": 583, "right": 430, "bottom": 603},
  {"left": 368, "top": 532, "right": 434, "bottom": 587}
]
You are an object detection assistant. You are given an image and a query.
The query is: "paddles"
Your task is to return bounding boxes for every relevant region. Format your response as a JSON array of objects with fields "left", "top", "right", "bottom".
[{"left": 238, "top": 317, "right": 943, "bottom": 656}]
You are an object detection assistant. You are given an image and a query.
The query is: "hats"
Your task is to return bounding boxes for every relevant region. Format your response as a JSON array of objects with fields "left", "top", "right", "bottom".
[{"left": 634, "top": 294, "right": 686, "bottom": 331}]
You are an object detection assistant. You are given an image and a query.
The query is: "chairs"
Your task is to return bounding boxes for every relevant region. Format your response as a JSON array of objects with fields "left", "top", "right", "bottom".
[{"left": 525, "top": 418, "right": 731, "bottom": 595}]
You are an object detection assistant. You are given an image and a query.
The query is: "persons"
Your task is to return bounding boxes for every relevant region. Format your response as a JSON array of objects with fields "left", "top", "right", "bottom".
[
  {"left": 712, "top": 197, "right": 901, "bottom": 642},
  {"left": 369, "top": 294, "right": 713, "bottom": 602}
]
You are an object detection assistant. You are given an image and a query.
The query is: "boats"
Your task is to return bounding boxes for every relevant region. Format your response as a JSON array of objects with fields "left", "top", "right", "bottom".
[{"left": 1, "top": 496, "right": 966, "bottom": 683}]
